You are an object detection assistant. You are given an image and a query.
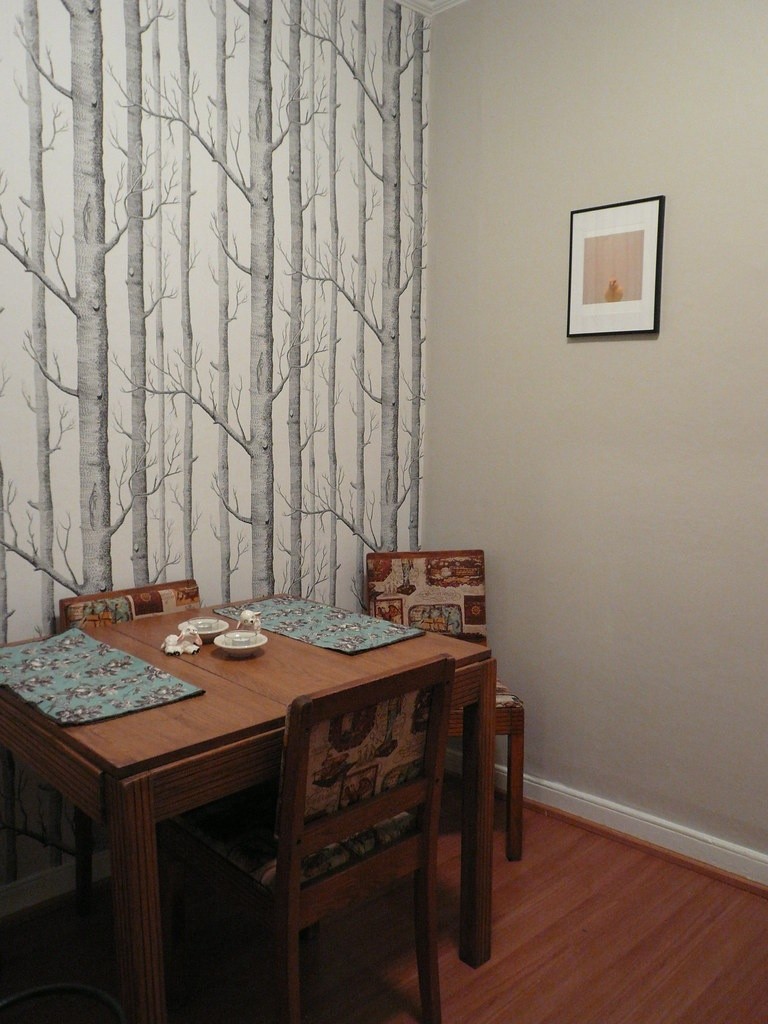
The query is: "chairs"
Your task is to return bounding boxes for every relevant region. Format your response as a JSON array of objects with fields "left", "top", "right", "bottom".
[
  {"left": 157, "top": 653, "right": 456, "bottom": 1024},
  {"left": 367, "top": 549, "right": 524, "bottom": 862},
  {"left": 58, "top": 579, "right": 202, "bottom": 914}
]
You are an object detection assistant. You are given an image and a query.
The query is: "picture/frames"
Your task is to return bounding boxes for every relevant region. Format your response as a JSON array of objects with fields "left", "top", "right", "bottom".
[{"left": 565, "top": 195, "right": 665, "bottom": 338}]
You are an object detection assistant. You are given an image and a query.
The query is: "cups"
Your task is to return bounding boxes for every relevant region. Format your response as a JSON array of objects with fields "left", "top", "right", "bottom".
[
  {"left": 187, "top": 616, "right": 219, "bottom": 632},
  {"left": 224, "top": 629, "right": 258, "bottom": 646}
]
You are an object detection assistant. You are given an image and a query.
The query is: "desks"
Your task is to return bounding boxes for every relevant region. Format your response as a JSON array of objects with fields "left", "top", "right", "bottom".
[{"left": 0, "top": 594, "right": 497, "bottom": 1024}]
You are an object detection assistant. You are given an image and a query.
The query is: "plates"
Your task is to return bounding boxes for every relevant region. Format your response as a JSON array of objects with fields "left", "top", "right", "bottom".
[
  {"left": 214, "top": 633, "right": 268, "bottom": 658},
  {"left": 178, "top": 618, "right": 229, "bottom": 644}
]
